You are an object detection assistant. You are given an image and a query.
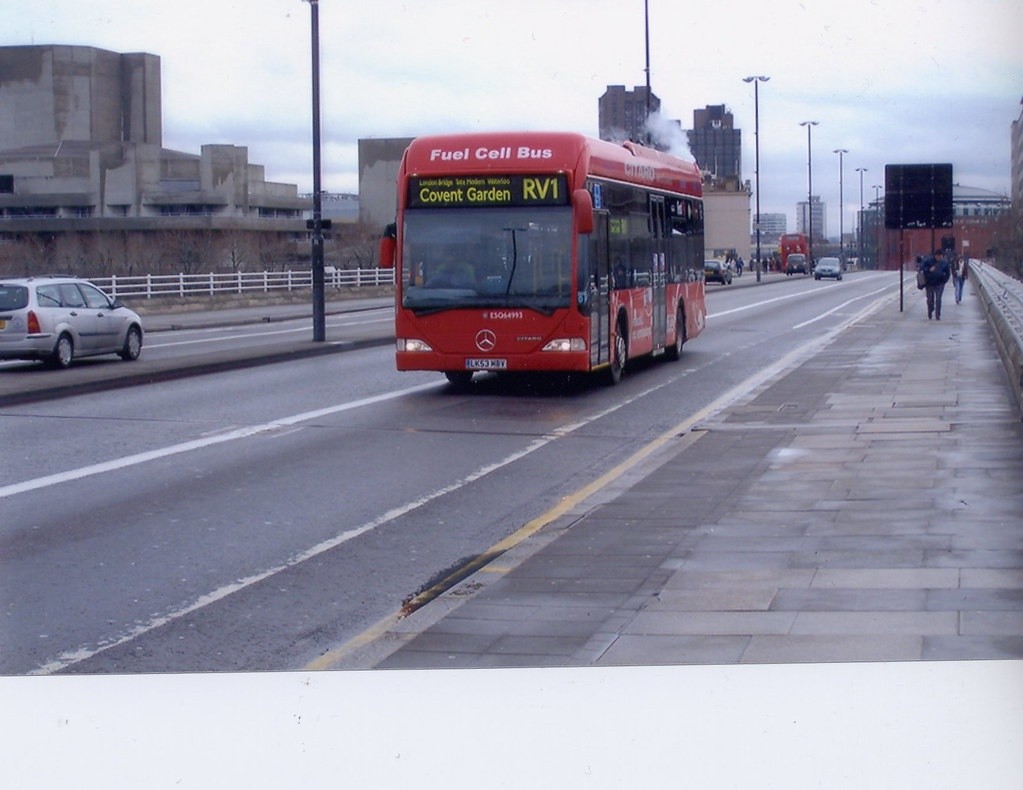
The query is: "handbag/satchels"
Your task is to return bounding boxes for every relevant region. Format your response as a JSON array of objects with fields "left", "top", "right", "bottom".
[{"left": 917, "top": 271, "right": 926, "bottom": 289}]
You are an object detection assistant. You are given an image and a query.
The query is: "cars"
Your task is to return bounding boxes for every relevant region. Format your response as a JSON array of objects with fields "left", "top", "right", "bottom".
[
  {"left": 815, "top": 256, "right": 843, "bottom": 281},
  {"left": 703, "top": 259, "right": 733, "bottom": 286},
  {"left": 786, "top": 253, "right": 808, "bottom": 277},
  {"left": 0, "top": 276, "right": 144, "bottom": 367}
]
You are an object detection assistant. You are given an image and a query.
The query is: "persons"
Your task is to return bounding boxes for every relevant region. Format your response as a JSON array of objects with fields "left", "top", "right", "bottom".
[
  {"left": 762, "top": 255, "right": 781, "bottom": 272},
  {"left": 424, "top": 254, "right": 488, "bottom": 294},
  {"left": 915, "top": 248, "right": 968, "bottom": 320},
  {"left": 749, "top": 257, "right": 754, "bottom": 271},
  {"left": 736, "top": 257, "right": 743, "bottom": 277}
]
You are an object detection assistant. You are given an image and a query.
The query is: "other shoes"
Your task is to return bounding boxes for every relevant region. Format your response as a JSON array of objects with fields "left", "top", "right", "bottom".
[
  {"left": 928, "top": 310, "right": 932, "bottom": 319},
  {"left": 936, "top": 312, "right": 940, "bottom": 320}
]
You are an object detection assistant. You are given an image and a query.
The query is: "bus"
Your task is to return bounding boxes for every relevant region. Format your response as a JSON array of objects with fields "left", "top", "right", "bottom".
[
  {"left": 778, "top": 232, "right": 815, "bottom": 273},
  {"left": 377, "top": 126, "right": 710, "bottom": 391}
]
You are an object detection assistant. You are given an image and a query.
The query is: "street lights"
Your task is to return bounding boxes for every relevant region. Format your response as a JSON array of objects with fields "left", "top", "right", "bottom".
[
  {"left": 743, "top": 74, "right": 770, "bottom": 283},
  {"left": 871, "top": 185, "right": 882, "bottom": 269},
  {"left": 834, "top": 149, "right": 848, "bottom": 271},
  {"left": 855, "top": 167, "right": 868, "bottom": 271},
  {"left": 798, "top": 120, "right": 820, "bottom": 277}
]
